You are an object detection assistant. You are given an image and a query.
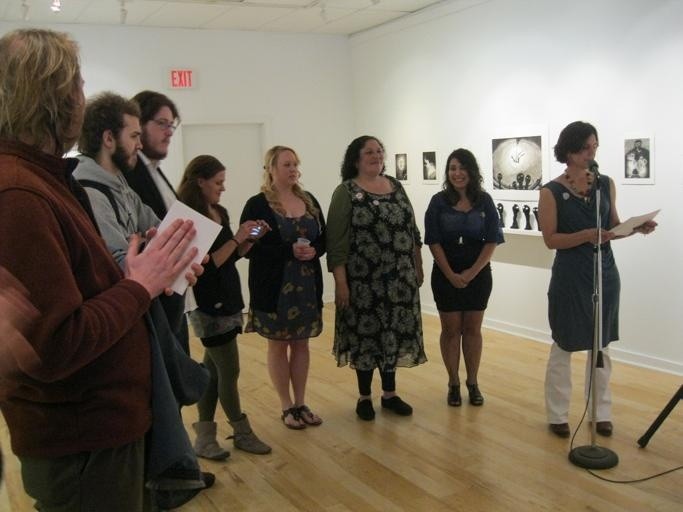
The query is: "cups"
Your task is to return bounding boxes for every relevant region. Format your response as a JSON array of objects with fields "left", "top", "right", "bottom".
[{"left": 297, "top": 238, "right": 311, "bottom": 247}]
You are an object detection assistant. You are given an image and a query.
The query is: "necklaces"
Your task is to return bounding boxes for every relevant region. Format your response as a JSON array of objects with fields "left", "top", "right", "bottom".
[{"left": 564, "top": 168, "right": 592, "bottom": 196}]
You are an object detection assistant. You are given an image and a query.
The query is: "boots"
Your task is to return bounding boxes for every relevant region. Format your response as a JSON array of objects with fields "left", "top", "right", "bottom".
[
  {"left": 191, "top": 420, "right": 230, "bottom": 460},
  {"left": 225, "top": 411, "right": 273, "bottom": 455}
]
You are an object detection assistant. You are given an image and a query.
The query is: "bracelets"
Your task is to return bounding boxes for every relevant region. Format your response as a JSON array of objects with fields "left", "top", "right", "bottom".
[{"left": 231, "top": 237, "right": 240, "bottom": 247}]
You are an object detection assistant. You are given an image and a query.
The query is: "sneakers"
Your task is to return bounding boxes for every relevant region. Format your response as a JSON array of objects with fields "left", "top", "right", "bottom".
[
  {"left": 355, "top": 398, "right": 377, "bottom": 422},
  {"left": 381, "top": 395, "right": 413, "bottom": 418}
]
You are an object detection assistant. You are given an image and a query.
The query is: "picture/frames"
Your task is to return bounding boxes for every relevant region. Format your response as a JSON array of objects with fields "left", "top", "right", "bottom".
[
  {"left": 491, "top": 201, "right": 545, "bottom": 237},
  {"left": 621, "top": 132, "right": 655, "bottom": 186},
  {"left": 420, "top": 148, "right": 440, "bottom": 184},
  {"left": 487, "top": 123, "right": 549, "bottom": 202},
  {"left": 393, "top": 151, "right": 411, "bottom": 185}
]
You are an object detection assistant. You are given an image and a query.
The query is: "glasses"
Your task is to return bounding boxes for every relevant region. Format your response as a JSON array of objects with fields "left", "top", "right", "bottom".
[{"left": 150, "top": 116, "right": 181, "bottom": 131}]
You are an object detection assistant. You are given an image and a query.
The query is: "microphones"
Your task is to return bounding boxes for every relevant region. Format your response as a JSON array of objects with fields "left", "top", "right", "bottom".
[{"left": 587, "top": 159, "right": 604, "bottom": 184}]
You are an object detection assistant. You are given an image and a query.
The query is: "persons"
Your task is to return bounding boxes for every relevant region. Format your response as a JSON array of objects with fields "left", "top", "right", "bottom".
[
  {"left": 238, "top": 146, "right": 327, "bottom": 430},
  {"left": 127, "top": 88, "right": 191, "bottom": 359},
  {"left": 424, "top": 148, "right": 505, "bottom": 406},
  {"left": 0, "top": 28, "right": 210, "bottom": 512},
  {"left": 173, "top": 154, "right": 271, "bottom": 460},
  {"left": 70, "top": 93, "right": 211, "bottom": 277},
  {"left": 538, "top": 121, "right": 658, "bottom": 438},
  {"left": 325, "top": 135, "right": 425, "bottom": 421}
]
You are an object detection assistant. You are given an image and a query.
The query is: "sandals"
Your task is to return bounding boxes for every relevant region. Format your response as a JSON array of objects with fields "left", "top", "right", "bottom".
[
  {"left": 299, "top": 404, "right": 323, "bottom": 425},
  {"left": 468, "top": 382, "right": 484, "bottom": 407},
  {"left": 446, "top": 381, "right": 462, "bottom": 406},
  {"left": 279, "top": 407, "right": 305, "bottom": 430}
]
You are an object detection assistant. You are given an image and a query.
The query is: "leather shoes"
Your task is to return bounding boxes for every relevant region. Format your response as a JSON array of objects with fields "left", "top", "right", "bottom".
[
  {"left": 551, "top": 421, "right": 570, "bottom": 438},
  {"left": 597, "top": 420, "right": 612, "bottom": 436}
]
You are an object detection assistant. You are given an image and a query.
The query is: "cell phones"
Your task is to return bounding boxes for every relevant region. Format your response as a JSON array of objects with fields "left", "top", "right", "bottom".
[{"left": 250, "top": 226, "right": 262, "bottom": 236}]
